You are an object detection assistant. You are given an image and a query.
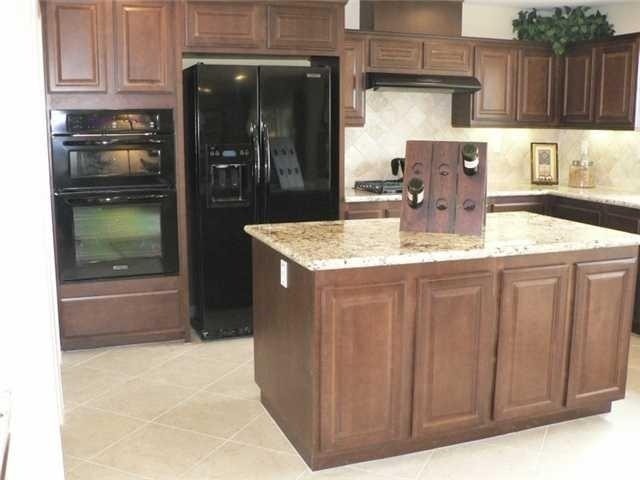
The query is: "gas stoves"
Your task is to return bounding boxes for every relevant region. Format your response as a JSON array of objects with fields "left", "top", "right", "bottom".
[{"left": 355, "top": 178, "right": 403, "bottom": 195}]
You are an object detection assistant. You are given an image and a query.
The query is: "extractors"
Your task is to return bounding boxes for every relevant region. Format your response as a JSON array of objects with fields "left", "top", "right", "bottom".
[{"left": 357, "top": 1, "right": 484, "bottom": 95}]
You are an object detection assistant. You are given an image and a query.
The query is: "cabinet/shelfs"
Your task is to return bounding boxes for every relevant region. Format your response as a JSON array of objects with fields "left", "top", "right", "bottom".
[
  {"left": 340, "top": 29, "right": 364, "bottom": 127},
  {"left": 179, "top": 0, "right": 346, "bottom": 53},
  {"left": 366, "top": 34, "right": 473, "bottom": 77},
  {"left": 559, "top": 31, "right": 640, "bottom": 131},
  {"left": 549, "top": 195, "right": 640, "bottom": 335},
  {"left": 42, "top": 0, "right": 174, "bottom": 111},
  {"left": 453, "top": 39, "right": 558, "bottom": 128}
]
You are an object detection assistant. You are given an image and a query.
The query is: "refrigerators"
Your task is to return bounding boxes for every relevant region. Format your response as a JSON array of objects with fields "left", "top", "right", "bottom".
[{"left": 182, "top": 62, "right": 337, "bottom": 340}]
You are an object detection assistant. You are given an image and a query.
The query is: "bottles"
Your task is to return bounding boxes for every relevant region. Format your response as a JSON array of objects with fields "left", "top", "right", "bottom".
[
  {"left": 461, "top": 143, "right": 482, "bottom": 176},
  {"left": 567, "top": 160, "right": 597, "bottom": 188},
  {"left": 406, "top": 176, "right": 426, "bottom": 209}
]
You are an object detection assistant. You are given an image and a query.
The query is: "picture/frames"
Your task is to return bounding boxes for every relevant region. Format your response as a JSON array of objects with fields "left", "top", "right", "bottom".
[{"left": 533, "top": 144, "right": 558, "bottom": 184}]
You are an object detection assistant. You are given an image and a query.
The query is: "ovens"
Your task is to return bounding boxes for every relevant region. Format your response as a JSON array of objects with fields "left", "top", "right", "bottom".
[{"left": 48, "top": 109, "right": 180, "bottom": 283}]
